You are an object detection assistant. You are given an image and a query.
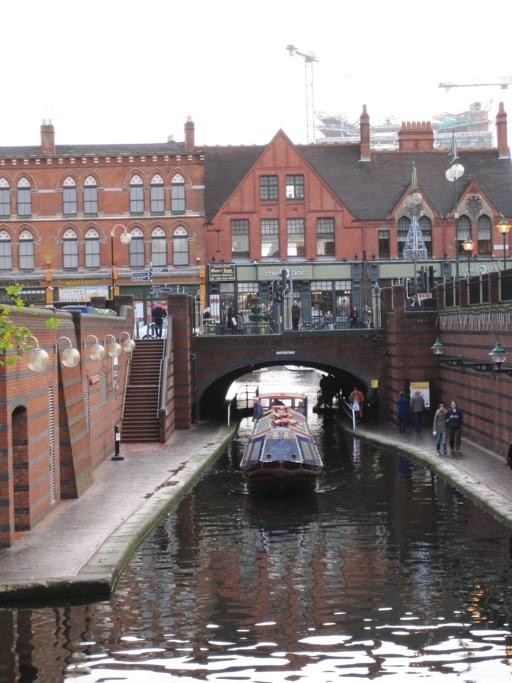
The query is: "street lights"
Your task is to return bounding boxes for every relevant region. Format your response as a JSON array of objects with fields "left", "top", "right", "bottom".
[
  {"left": 110, "top": 224, "right": 132, "bottom": 319},
  {"left": 495, "top": 215, "right": 511, "bottom": 299},
  {"left": 445, "top": 127, "right": 465, "bottom": 306},
  {"left": 402, "top": 160, "right": 427, "bottom": 296},
  {"left": 461, "top": 236, "right": 475, "bottom": 305}
]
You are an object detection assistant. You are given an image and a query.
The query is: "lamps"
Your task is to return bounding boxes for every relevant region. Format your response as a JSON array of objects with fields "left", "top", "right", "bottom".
[
  {"left": 430, "top": 336, "right": 463, "bottom": 368},
  {"left": 25, "top": 331, "right": 136, "bottom": 373},
  {"left": 488, "top": 340, "right": 511, "bottom": 377}
]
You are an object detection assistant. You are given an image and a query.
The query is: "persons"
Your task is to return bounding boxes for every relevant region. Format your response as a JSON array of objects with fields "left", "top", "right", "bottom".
[
  {"left": 410, "top": 392, "right": 425, "bottom": 430},
  {"left": 224, "top": 297, "right": 360, "bottom": 330},
  {"left": 349, "top": 387, "right": 364, "bottom": 417},
  {"left": 152, "top": 302, "right": 167, "bottom": 339},
  {"left": 320, "top": 374, "right": 338, "bottom": 408},
  {"left": 269, "top": 399, "right": 285, "bottom": 408},
  {"left": 396, "top": 390, "right": 409, "bottom": 433},
  {"left": 252, "top": 398, "right": 264, "bottom": 427},
  {"left": 447, "top": 400, "right": 463, "bottom": 454},
  {"left": 433, "top": 402, "right": 449, "bottom": 454},
  {"left": 505, "top": 441, "right": 512, "bottom": 469}
]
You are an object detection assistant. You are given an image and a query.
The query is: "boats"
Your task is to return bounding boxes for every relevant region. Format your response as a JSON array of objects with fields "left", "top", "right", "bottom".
[
  {"left": 239, "top": 392, "right": 324, "bottom": 487},
  {"left": 312, "top": 102, "right": 493, "bottom": 152},
  {"left": 245, "top": 484, "right": 320, "bottom": 539}
]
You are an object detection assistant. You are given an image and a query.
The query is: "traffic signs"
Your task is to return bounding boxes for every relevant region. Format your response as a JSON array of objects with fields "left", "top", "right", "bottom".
[
  {"left": 151, "top": 267, "right": 175, "bottom": 272},
  {"left": 130, "top": 275, "right": 149, "bottom": 280},
  {"left": 132, "top": 272, "right": 148, "bottom": 275},
  {"left": 149, "top": 287, "right": 173, "bottom": 293}
]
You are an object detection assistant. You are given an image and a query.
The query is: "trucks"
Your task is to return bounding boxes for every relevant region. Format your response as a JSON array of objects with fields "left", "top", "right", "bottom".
[{"left": 198, "top": 308, "right": 275, "bottom": 334}]
